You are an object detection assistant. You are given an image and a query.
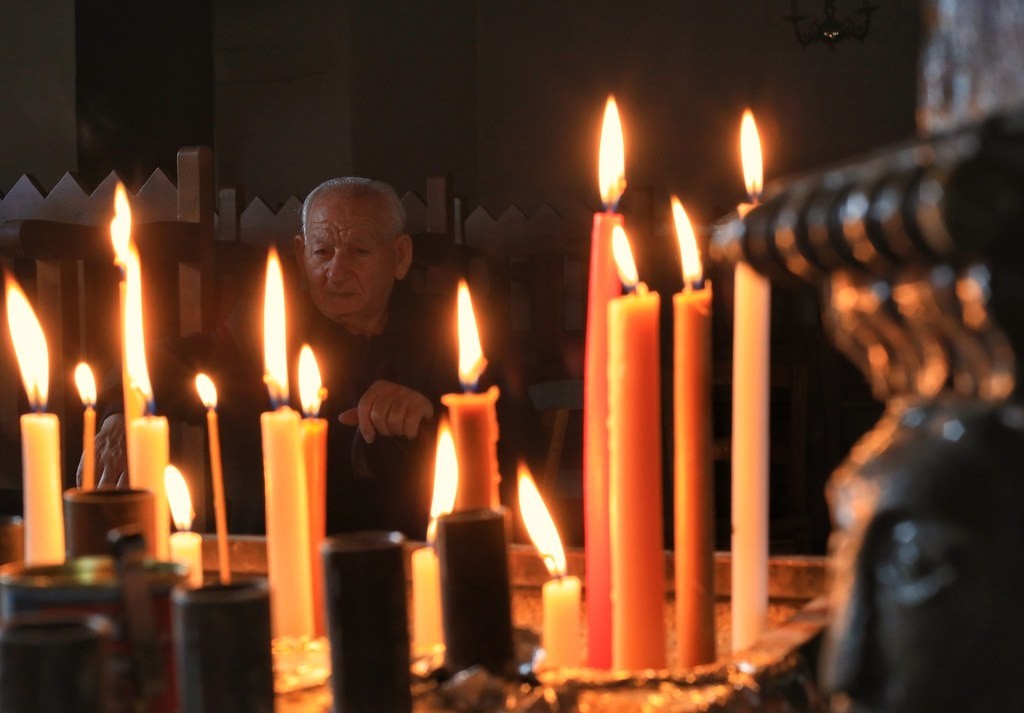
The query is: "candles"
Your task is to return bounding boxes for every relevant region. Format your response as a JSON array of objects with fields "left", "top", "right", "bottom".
[
  {"left": 676, "top": 288, "right": 715, "bottom": 661},
  {"left": 126, "top": 418, "right": 170, "bottom": 561},
  {"left": 206, "top": 410, "right": 232, "bottom": 585},
  {"left": 585, "top": 215, "right": 664, "bottom": 671},
  {"left": 20, "top": 413, "right": 67, "bottom": 565},
  {"left": 172, "top": 533, "right": 202, "bottom": 587},
  {"left": 82, "top": 410, "right": 95, "bottom": 489},
  {"left": 262, "top": 411, "right": 328, "bottom": 642},
  {"left": 407, "top": 550, "right": 442, "bottom": 645},
  {"left": 543, "top": 575, "right": 582, "bottom": 666},
  {"left": 730, "top": 205, "right": 769, "bottom": 655},
  {"left": 440, "top": 386, "right": 500, "bottom": 511}
]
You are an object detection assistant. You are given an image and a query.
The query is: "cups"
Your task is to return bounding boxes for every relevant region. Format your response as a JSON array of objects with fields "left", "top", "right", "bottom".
[{"left": 64, "top": 487, "right": 155, "bottom": 562}]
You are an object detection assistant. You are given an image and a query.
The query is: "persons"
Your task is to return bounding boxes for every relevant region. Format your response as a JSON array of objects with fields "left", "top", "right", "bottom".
[{"left": 74, "top": 175, "right": 512, "bottom": 536}]
